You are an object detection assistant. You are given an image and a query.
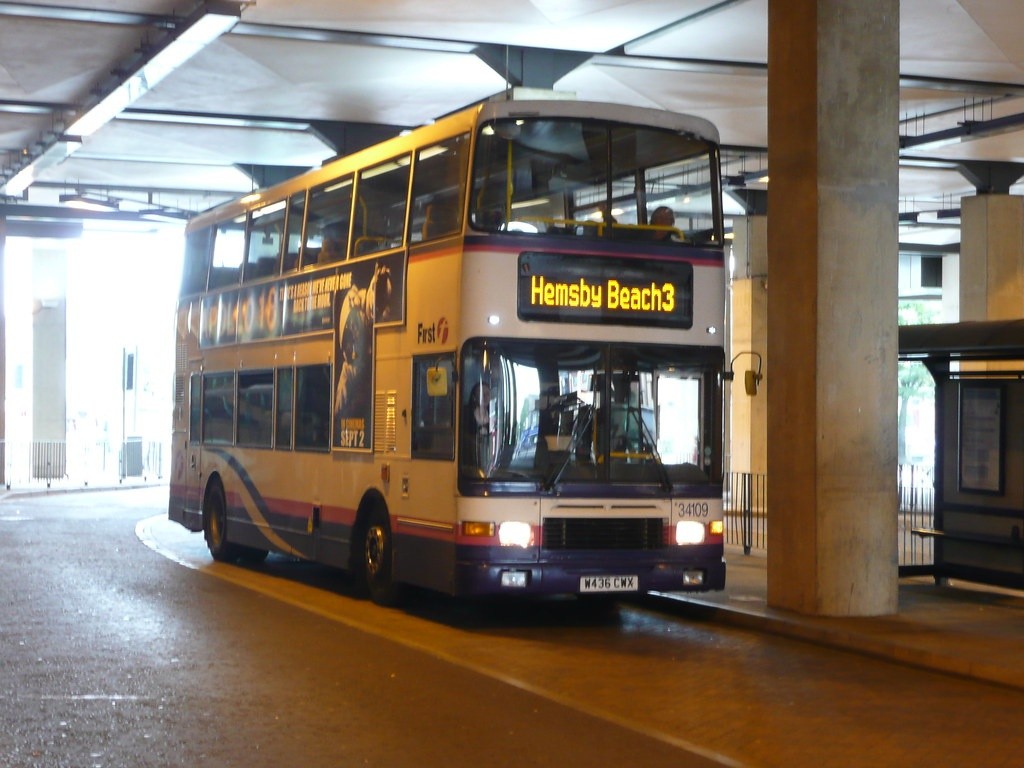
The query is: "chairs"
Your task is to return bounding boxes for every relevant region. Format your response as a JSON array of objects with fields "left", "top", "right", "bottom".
[{"left": 236, "top": 206, "right": 675, "bottom": 281}]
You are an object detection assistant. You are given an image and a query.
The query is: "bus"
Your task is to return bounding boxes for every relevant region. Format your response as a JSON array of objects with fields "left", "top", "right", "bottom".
[{"left": 168, "top": 85, "right": 763, "bottom": 607}]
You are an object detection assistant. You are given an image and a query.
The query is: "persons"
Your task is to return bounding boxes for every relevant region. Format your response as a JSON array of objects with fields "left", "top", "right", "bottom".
[
  {"left": 462, "top": 381, "right": 497, "bottom": 462},
  {"left": 651, "top": 206, "right": 675, "bottom": 240}
]
